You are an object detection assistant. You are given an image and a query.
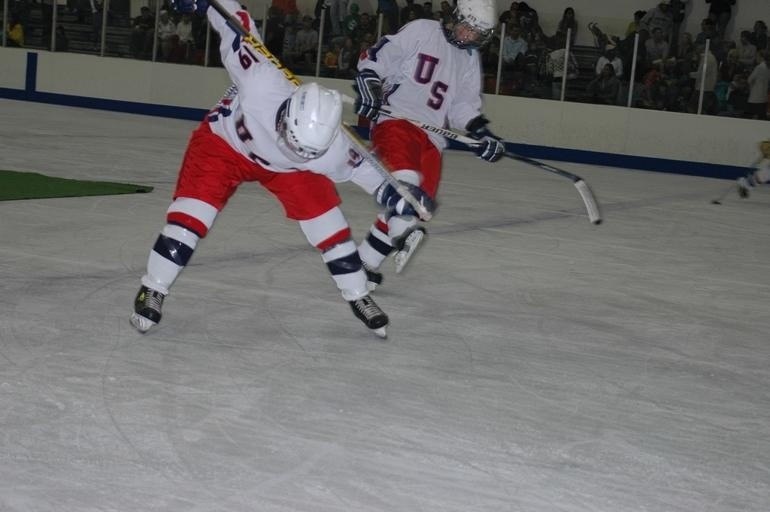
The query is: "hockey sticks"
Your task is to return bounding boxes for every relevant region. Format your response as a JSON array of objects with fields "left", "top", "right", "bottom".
[
  {"left": 341, "top": 95, "right": 602, "bottom": 225},
  {"left": 712, "top": 154, "right": 765, "bottom": 205}
]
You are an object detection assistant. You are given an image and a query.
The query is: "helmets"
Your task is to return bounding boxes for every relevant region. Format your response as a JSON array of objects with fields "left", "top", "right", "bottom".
[
  {"left": 446, "top": 1, "right": 497, "bottom": 49},
  {"left": 275, "top": 81, "right": 343, "bottom": 164}
]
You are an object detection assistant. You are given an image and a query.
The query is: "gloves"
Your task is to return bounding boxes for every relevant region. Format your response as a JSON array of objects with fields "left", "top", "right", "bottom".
[
  {"left": 465, "top": 114, "right": 504, "bottom": 164},
  {"left": 377, "top": 177, "right": 433, "bottom": 222},
  {"left": 353, "top": 69, "right": 383, "bottom": 122}
]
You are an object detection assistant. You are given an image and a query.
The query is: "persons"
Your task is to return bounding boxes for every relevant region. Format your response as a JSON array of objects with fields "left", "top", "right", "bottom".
[
  {"left": 352, "top": 0, "right": 510, "bottom": 286},
  {"left": 1, "top": 2, "right": 225, "bottom": 67},
  {"left": 737, "top": 139, "right": 768, "bottom": 201},
  {"left": 132, "top": 0, "right": 435, "bottom": 330},
  {"left": 480, "top": 1, "right": 769, "bottom": 119},
  {"left": 257, "top": 2, "right": 456, "bottom": 78}
]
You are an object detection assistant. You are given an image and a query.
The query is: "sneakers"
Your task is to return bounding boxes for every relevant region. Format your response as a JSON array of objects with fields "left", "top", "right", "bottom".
[
  {"left": 135, "top": 286, "right": 164, "bottom": 325},
  {"left": 390, "top": 226, "right": 425, "bottom": 262},
  {"left": 350, "top": 294, "right": 388, "bottom": 330},
  {"left": 736, "top": 173, "right": 759, "bottom": 201},
  {"left": 361, "top": 259, "right": 382, "bottom": 285}
]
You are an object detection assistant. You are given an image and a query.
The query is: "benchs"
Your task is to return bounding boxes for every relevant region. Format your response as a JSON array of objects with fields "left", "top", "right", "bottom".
[
  {"left": 0, "top": 4, "right": 138, "bottom": 60},
  {"left": 567, "top": 44, "right": 602, "bottom": 94}
]
